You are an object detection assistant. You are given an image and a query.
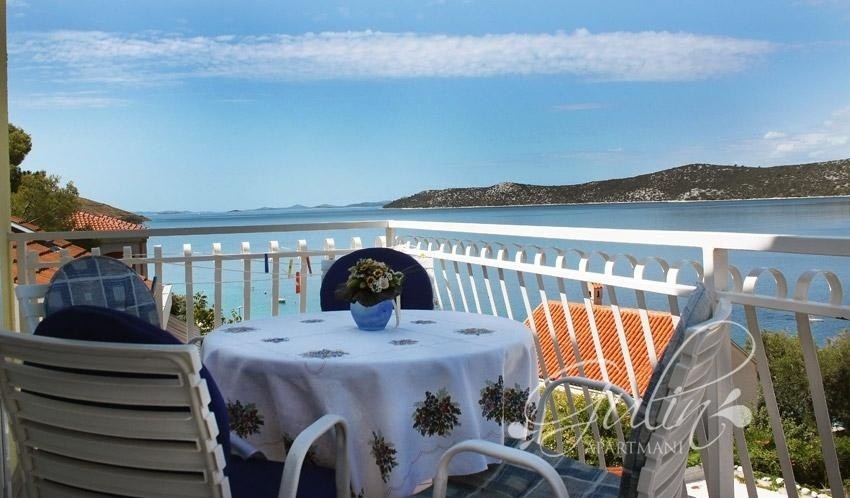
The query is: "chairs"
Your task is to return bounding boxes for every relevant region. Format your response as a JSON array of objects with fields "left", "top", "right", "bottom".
[{"left": 0, "top": 248, "right": 732, "bottom": 498}]
